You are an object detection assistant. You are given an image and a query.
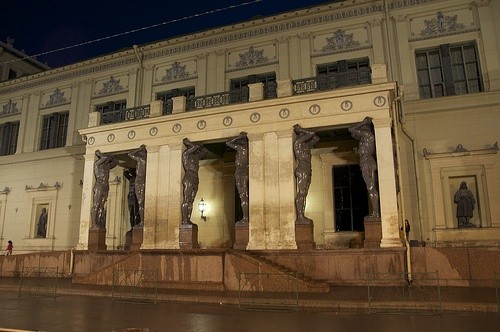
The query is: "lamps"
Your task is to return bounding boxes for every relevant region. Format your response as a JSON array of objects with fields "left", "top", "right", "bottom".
[{"left": 197, "top": 198, "right": 207, "bottom": 222}]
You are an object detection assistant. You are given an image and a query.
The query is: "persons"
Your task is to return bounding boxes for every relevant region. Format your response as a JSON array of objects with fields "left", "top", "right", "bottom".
[
  {"left": 292, "top": 124, "right": 321, "bottom": 225},
  {"left": 128, "top": 144, "right": 147, "bottom": 231},
  {"left": 181, "top": 137, "right": 203, "bottom": 226},
  {"left": 454, "top": 182, "right": 476, "bottom": 229},
  {"left": 401, "top": 219, "right": 410, "bottom": 247},
  {"left": 349, "top": 116, "right": 381, "bottom": 218},
  {"left": 91, "top": 150, "right": 119, "bottom": 229},
  {"left": 34, "top": 207, "right": 48, "bottom": 239},
  {"left": 123, "top": 166, "right": 140, "bottom": 231},
  {"left": 226, "top": 131, "right": 250, "bottom": 226},
  {"left": 5, "top": 240, "right": 14, "bottom": 256}
]
